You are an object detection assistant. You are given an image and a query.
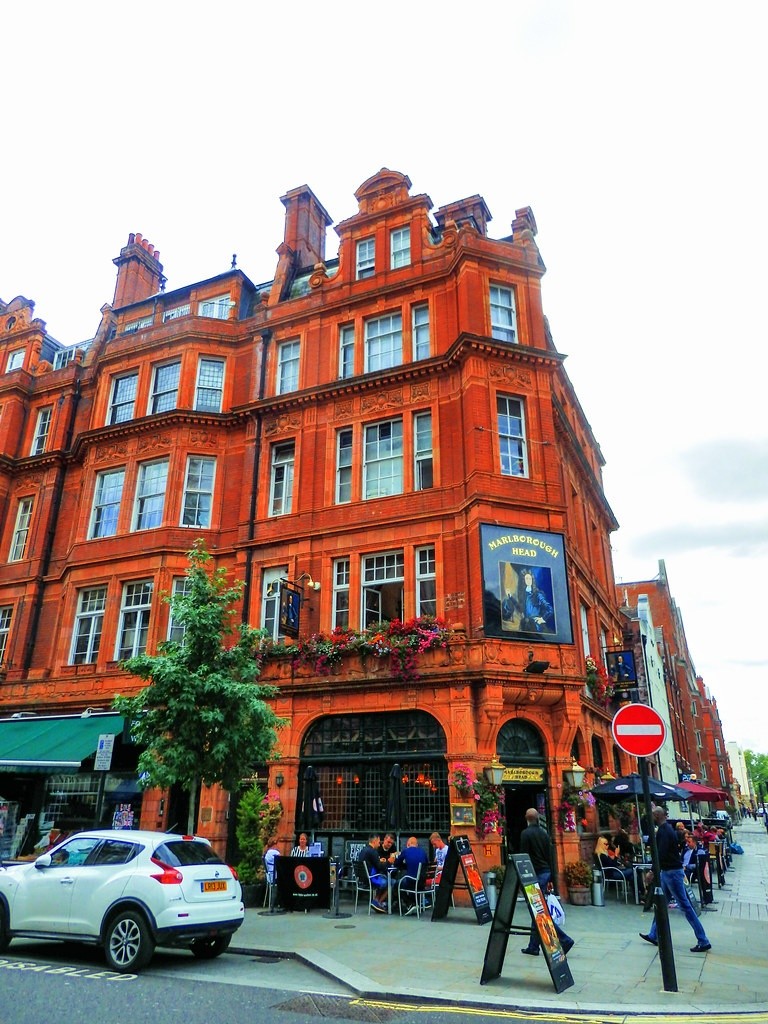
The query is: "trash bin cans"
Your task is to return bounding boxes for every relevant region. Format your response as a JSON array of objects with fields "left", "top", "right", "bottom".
[
  {"left": 483, "top": 871, "right": 498, "bottom": 911},
  {"left": 592, "top": 869, "right": 606, "bottom": 907}
]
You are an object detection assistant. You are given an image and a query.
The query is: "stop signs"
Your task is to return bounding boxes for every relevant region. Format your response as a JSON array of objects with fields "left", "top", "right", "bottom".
[{"left": 612, "top": 703, "right": 668, "bottom": 758}]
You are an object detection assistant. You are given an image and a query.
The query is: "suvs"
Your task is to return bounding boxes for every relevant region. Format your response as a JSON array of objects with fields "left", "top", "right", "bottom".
[{"left": 0, "top": 830, "right": 245, "bottom": 974}]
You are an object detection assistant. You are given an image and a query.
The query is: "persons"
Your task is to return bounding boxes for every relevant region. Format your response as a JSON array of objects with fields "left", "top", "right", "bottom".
[
  {"left": 359, "top": 834, "right": 396, "bottom": 913},
  {"left": 520, "top": 807, "right": 577, "bottom": 958},
  {"left": 640, "top": 807, "right": 713, "bottom": 953},
  {"left": 289, "top": 832, "right": 312, "bottom": 859},
  {"left": 395, "top": 837, "right": 429, "bottom": 916},
  {"left": 422, "top": 831, "right": 450, "bottom": 886},
  {"left": 592, "top": 819, "right": 728, "bottom": 911},
  {"left": 377, "top": 834, "right": 397, "bottom": 880},
  {"left": 264, "top": 837, "right": 284, "bottom": 886},
  {"left": 743, "top": 807, "right": 752, "bottom": 819}
]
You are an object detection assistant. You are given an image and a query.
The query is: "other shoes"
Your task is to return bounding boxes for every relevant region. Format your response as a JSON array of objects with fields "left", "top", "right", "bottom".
[
  {"left": 690, "top": 943, "right": 711, "bottom": 952},
  {"left": 371, "top": 900, "right": 388, "bottom": 914},
  {"left": 639, "top": 933, "right": 658, "bottom": 946},
  {"left": 564, "top": 941, "right": 574, "bottom": 955},
  {"left": 521, "top": 948, "right": 539, "bottom": 956}
]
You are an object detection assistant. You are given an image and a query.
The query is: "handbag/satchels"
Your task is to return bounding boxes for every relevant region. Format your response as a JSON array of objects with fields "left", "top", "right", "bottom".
[{"left": 547, "top": 892, "right": 566, "bottom": 925}]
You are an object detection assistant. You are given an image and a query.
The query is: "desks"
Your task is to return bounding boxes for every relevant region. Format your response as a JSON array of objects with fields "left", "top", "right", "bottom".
[{"left": 633, "top": 864, "right": 651, "bottom": 905}]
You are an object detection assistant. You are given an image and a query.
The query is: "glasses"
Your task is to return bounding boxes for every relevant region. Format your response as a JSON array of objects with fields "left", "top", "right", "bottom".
[{"left": 604, "top": 843, "right": 609, "bottom": 846}]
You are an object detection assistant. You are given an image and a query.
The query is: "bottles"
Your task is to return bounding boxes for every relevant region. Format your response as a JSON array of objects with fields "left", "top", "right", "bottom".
[{"left": 646, "top": 846, "right": 651, "bottom": 857}]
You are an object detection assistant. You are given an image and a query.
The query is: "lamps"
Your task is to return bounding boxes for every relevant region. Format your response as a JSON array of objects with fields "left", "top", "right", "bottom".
[
  {"left": 403, "top": 763, "right": 438, "bottom": 791},
  {"left": 562, "top": 757, "right": 586, "bottom": 787},
  {"left": 522, "top": 658, "right": 550, "bottom": 675},
  {"left": 11, "top": 711, "right": 35, "bottom": 718},
  {"left": 275, "top": 773, "right": 284, "bottom": 788},
  {"left": 81, "top": 708, "right": 103, "bottom": 719},
  {"left": 265, "top": 572, "right": 315, "bottom": 598},
  {"left": 482, "top": 752, "right": 504, "bottom": 786},
  {"left": 601, "top": 768, "right": 615, "bottom": 782}
]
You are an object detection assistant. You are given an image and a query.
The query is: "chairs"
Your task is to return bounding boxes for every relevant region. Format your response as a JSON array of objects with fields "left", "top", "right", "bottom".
[
  {"left": 262, "top": 857, "right": 458, "bottom": 921},
  {"left": 597, "top": 838, "right": 728, "bottom": 905}
]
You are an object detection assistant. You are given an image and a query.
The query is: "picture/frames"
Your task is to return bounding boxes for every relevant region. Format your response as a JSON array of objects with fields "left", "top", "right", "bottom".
[{"left": 450, "top": 803, "right": 476, "bottom": 827}]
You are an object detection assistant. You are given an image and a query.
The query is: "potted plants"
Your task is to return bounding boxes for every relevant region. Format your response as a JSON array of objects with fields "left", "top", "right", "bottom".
[{"left": 566, "top": 861, "right": 596, "bottom": 907}]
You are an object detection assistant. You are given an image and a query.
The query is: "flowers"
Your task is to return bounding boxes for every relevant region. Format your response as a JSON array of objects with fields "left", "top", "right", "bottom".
[
  {"left": 556, "top": 786, "right": 599, "bottom": 833},
  {"left": 448, "top": 766, "right": 505, "bottom": 841},
  {"left": 586, "top": 655, "right": 615, "bottom": 709},
  {"left": 255, "top": 612, "right": 450, "bottom": 681}
]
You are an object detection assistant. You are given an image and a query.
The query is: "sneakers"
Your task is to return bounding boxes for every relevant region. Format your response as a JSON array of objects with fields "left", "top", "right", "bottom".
[
  {"left": 405, "top": 904, "right": 419, "bottom": 916},
  {"left": 420, "top": 901, "right": 431, "bottom": 908}
]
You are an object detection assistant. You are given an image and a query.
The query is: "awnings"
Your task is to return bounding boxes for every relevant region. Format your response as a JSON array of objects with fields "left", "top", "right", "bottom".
[{"left": 0, "top": 708, "right": 150, "bottom": 774}]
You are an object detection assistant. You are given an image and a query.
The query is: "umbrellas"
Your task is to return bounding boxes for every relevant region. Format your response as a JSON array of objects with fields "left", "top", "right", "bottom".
[
  {"left": 585, "top": 768, "right": 693, "bottom": 868},
  {"left": 301, "top": 764, "right": 325, "bottom": 845},
  {"left": 379, "top": 761, "right": 409, "bottom": 849},
  {"left": 672, "top": 782, "right": 728, "bottom": 831}
]
